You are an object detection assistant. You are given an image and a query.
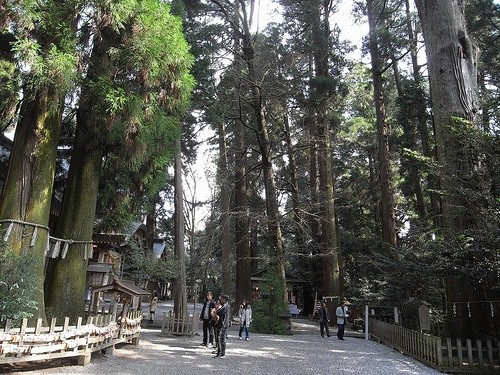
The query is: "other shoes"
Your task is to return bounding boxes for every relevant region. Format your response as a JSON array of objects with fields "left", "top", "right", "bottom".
[
  {"left": 212, "top": 351, "right": 218, "bottom": 353},
  {"left": 240, "top": 337, "right": 242, "bottom": 339},
  {"left": 245, "top": 337, "right": 249, "bottom": 341},
  {"left": 214, "top": 356, "right": 225, "bottom": 358},
  {"left": 202, "top": 342, "right": 207, "bottom": 346}
]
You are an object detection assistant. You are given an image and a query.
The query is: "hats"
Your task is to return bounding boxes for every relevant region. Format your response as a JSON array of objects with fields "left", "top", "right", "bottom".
[{"left": 221, "top": 295, "right": 229, "bottom": 303}]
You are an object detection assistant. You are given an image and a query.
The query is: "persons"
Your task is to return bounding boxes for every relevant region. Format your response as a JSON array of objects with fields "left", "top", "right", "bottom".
[
  {"left": 211, "top": 293, "right": 231, "bottom": 359},
  {"left": 149, "top": 290, "right": 159, "bottom": 322},
  {"left": 199, "top": 291, "right": 215, "bottom": 346},
  {"left": 318, "top": 303, "right": 331, "bottom": 338},
  {"left": 336, "top": 302, "right": 349, "bottom": 340},
  {"left": 238, "top": 299, "right": 252, "bottom": 341}
]
